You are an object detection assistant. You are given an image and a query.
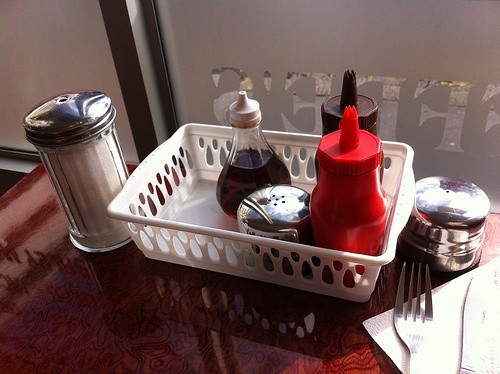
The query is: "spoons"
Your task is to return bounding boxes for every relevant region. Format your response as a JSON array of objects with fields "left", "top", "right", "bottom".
[{"left": 393, "top": 262, "right": 434, "bottom": 374}]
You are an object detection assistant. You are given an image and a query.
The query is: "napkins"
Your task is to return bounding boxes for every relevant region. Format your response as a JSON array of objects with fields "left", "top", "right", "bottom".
[{"left": 362, "top": 258, "right": 500, "bottom": 374}]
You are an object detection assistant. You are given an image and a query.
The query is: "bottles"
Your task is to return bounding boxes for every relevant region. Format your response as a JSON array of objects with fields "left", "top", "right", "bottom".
[
  {"left": 315, "top": 69, "right": 383, "bottom": 185},
  {"left": 310, "top": 105, "right": 387, "bottom": 286},
  {"left": 216, "top": 91, "right": 292, "bottom": 218},
  {"left": 23, "top": 89, "right": 133, "bottom": 253}
]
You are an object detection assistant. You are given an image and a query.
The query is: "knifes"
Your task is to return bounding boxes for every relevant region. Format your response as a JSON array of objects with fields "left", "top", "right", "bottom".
[{"left": 459, "top": 273, "right": 486, "bottom": 374}]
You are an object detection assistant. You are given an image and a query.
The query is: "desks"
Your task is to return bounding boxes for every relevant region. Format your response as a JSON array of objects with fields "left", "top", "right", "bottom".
[{"left": 0, "top": 159, "right": 500, "bottom": 374}]
